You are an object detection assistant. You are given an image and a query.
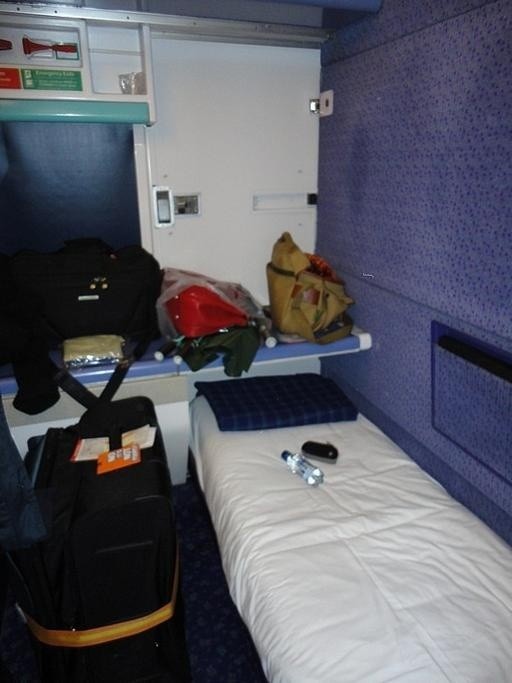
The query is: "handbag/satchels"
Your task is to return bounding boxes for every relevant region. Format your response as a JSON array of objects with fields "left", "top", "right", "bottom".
[
  {"left": 267, "top": 232, "right": 354, "bottom": 344},
  {"left": 1, "top": 238, "right": 161, "bottom": 358},
  {"left": 163, "top": 269, "right": 259, "bottom": 338}
]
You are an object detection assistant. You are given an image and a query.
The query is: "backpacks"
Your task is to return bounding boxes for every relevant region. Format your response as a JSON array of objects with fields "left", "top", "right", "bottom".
[{"left": 14, "top": 395, "right": 179, "bottom": 631}]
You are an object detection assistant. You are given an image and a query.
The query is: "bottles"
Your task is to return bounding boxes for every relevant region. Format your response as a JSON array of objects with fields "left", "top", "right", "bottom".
[{"left": 282, "top": 450, "right": 325, "bottom": 490}]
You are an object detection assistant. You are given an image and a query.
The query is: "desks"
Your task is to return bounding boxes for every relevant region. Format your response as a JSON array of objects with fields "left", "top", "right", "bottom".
[{"left": 0, "top": 303, "right": 373, "bottom": 410}]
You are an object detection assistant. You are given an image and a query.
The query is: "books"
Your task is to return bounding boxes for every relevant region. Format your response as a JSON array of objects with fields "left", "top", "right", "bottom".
[
  {"left": 70, "top": 436, "right": 110, "bottom": 462},
  {"left": 121, "top": 424, "right": 157, "bottom": 449},
  {"left": 96, "top": 442, "right": 142, "bottom": 474}
]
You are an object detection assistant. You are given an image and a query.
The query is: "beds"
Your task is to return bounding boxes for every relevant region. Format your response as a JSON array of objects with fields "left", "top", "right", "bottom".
[{"left": 188, "top": 394, "right": 512, "bottom": 682}]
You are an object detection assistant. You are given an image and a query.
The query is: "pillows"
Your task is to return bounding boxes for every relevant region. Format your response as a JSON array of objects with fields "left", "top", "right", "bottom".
[{"left": 193, "top": 373, "right": 359, "bottom": 432}]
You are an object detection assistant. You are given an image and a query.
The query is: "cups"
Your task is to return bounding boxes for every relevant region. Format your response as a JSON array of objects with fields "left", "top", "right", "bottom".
[{"left": 120, "top": 72, "right": 145, "bottom": 97}]
